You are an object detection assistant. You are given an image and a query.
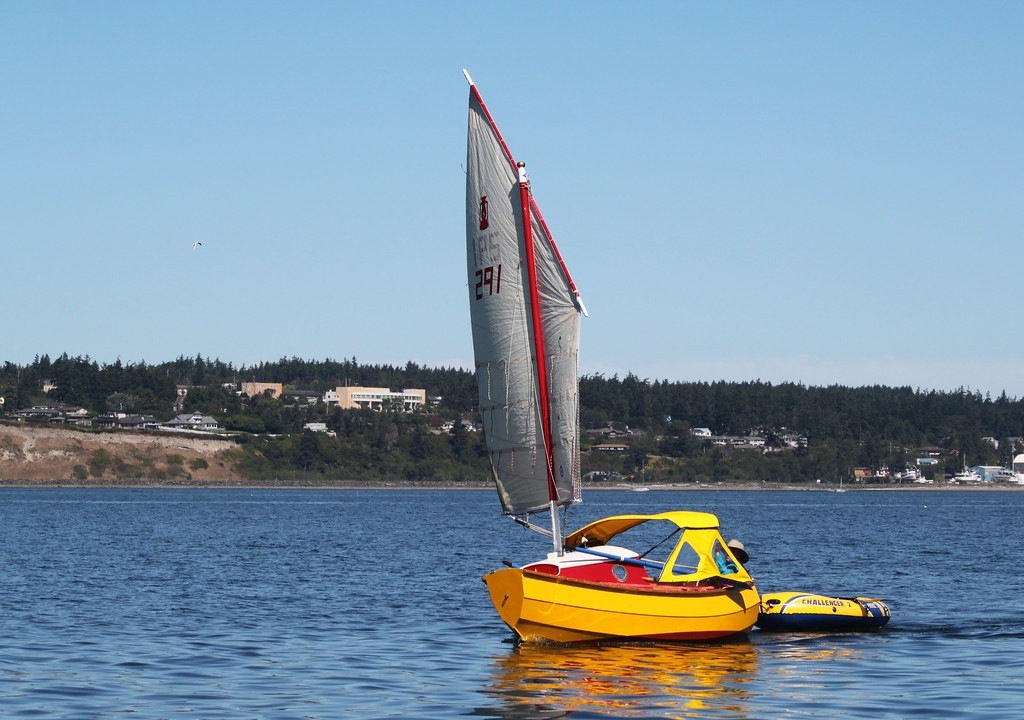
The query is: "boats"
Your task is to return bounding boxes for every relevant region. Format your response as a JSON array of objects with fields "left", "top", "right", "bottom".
[{"left": 760, "top": 585, "right": 891, "bottom": 632}]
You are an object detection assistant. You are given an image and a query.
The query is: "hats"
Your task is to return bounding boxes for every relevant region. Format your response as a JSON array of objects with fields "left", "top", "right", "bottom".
[{"left": 721, "top": 540, "right": 749, "bottom": 563}]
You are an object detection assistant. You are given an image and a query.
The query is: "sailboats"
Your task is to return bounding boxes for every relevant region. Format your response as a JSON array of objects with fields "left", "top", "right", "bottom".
[{"left": 459, "top": 68, "right": 761, "bottom": 644}]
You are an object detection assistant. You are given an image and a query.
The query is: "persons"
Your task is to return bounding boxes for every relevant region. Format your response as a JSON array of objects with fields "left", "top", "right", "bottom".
[
  {"left": 714, "top": 539, "right": 749, "bottom": 573},
  {"left": 581, "top": 534, "right": 603, "bottom": 547}
]
